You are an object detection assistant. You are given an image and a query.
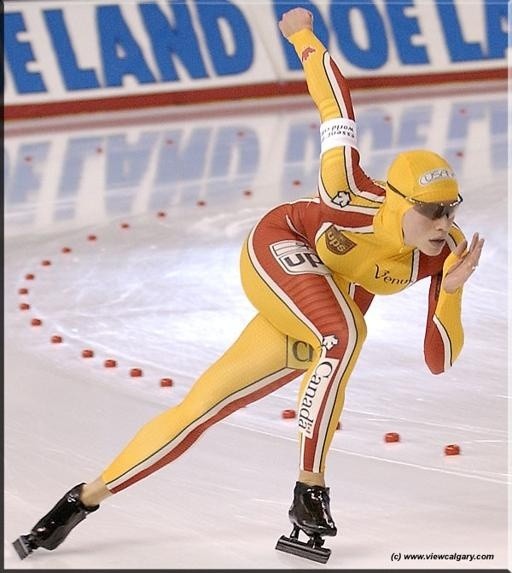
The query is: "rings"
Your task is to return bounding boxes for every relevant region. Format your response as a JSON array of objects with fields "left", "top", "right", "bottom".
[{"left": 470, "top": 265, "right": 479, "bottom": 270}]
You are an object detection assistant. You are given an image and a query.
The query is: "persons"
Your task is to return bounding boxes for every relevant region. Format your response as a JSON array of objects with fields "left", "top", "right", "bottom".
[{"left": 30, "top": 7, "right": 485, "bottom": 549}]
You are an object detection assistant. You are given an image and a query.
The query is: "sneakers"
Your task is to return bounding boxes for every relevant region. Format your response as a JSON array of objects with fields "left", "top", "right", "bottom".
[
  {"left": 30, "top": 483, "right": 100, "bottom": 550},
  {"left": 289, "top": 480, "right": 337, "bottom": 537}
]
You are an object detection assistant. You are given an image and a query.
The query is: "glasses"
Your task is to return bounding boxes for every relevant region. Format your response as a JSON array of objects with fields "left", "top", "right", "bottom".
[{"left": 386, "top": 179, "right": 463, "bottom": 220}]
